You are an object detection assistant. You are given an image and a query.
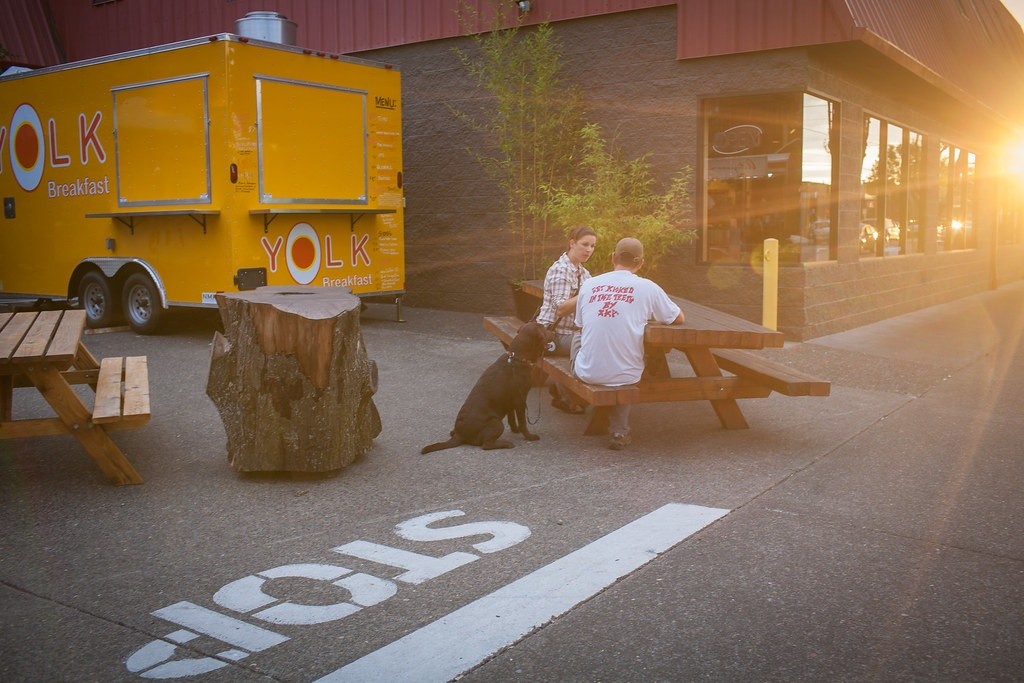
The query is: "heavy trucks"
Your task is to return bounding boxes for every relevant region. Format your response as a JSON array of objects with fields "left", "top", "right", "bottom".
[{"left": 0, "top": 9, "right": 409, "bottom": 331}]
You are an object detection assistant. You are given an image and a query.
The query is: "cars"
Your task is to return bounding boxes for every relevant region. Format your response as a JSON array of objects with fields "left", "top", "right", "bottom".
[{"left": 809, "top": 217, "right": 971, "bottom": 256}]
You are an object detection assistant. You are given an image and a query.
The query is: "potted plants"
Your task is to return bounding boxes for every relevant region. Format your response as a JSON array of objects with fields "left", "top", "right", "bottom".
[{"left": 446, "top": 1, "right": 703, "bottom": 319}]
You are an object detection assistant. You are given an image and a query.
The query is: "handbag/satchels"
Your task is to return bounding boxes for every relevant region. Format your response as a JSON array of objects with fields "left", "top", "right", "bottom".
[{"left": 529, "top": 319, "right": 557, "bottom": 356}]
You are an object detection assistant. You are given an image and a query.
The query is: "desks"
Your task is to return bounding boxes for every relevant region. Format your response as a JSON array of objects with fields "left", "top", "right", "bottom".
[
  {"left": 0, "top": 310, "right": 147, "bottom": 486},
  {"left": 522, "top": 279, "right": 784, "bottom": 437}
]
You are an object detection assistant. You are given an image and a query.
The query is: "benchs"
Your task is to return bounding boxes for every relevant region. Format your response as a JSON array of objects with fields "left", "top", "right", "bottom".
[
  {"left": 92, "top": 355, "right": 151, "bottom": 424},
  {"left": 483, "top": 314, "right": 640, "bottom": 407},
  {"left": 711, "top": 349, "right": 831, "bottom": 398}
]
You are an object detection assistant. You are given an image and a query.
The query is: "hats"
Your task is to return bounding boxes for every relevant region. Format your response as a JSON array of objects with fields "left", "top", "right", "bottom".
[{"left": 615, "top": 237, "right": 643, "bottom": 263}]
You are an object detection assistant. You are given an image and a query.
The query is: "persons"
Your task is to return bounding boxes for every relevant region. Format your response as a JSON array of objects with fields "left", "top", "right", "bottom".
[
  {"left": 570, "top": 236, "right": 685, "bottom": 450},
  {"left": 535, "top": 225, "right": 597, "bottom": 413}
]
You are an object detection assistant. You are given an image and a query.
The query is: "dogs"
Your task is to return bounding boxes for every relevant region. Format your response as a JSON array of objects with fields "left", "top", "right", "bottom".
[{"left": 421, "top": 323, "right": 558, "bottom": 455}]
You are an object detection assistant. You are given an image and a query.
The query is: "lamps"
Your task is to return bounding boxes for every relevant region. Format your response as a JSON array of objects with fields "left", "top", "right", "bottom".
[{"left": 515, "top": 0, "right": 534, "bottom": 15}]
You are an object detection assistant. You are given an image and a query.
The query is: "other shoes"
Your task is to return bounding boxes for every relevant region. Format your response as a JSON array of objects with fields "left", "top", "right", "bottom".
[
  {"left": 548, "top": 385, "right": 559, "bottom": 398},
  {"left": 551, "top": 397, "right": 584, "bottom": 414},
  {"left": 610, "top": 429, "right": 632, "bottom": 450}
]
